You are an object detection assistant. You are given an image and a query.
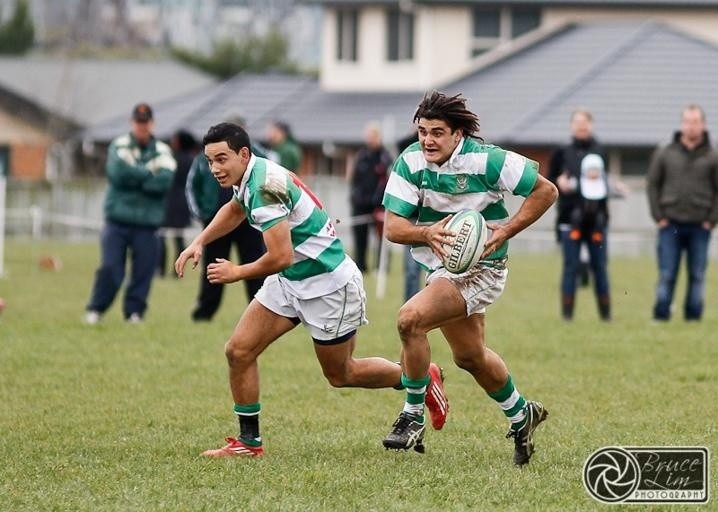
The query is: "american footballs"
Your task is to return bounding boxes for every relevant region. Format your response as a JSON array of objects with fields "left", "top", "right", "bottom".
[{"left": 440, "top": 210, "right": 488, "bottom": 273}]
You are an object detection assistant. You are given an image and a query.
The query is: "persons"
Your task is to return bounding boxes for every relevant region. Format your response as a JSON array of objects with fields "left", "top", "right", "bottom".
[
  {"left": 546, "top": 106, "right": 633, "bottom": 325},
  {"left": 381, "top": 89, "right": 560, "bottom": 466},
  {"left": 155, "top": 106, "right": 303, "bottom": 321},
  {"left": 644, "top": 104, "right": 717, "bottom": 322},
  {"left": 83, "top": 103, "right": 177, "bottom": 324},
  {"left": 174, "top": 122, "right": 449, "bottom": 458},
  {"left": 348, "top": 122, "right": 423, "bottom": 302}
]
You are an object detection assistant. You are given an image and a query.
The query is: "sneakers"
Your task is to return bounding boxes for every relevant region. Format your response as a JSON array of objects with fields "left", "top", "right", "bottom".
[
  {"left": 506, "top": 401, "right": 547, "bottom": 465},
  {"left": 199, "top": 438, "right": 263, "bottom": 459},
  {"left": 425, "top": 363, "right": 448, "bottom": 430},
  {"left": 382, "top": 411, "right": 424, "bottom": 452}
]
